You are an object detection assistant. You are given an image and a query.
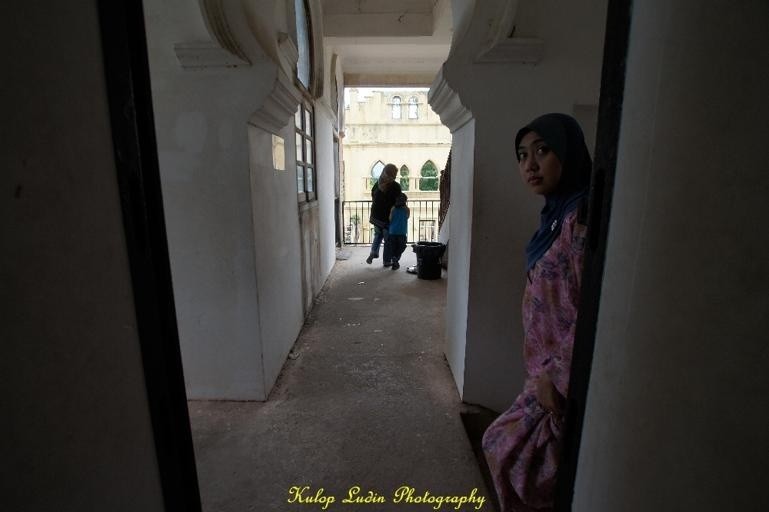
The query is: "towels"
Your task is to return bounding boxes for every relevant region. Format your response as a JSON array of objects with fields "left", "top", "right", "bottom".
[{"left": 412, "top": 242, "right": 447, "bottom": 280}]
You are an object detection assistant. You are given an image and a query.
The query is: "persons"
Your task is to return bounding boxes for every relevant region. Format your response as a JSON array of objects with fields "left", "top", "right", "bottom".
[
  {"left": 365, "top": 163, "right": 402, "bottom": 266},
  {"left": 483, "top": 112, "right": 593, "bottom": 512},
  {"left": 389, "top": 193, "right": 410, "bottom": 270}
]
